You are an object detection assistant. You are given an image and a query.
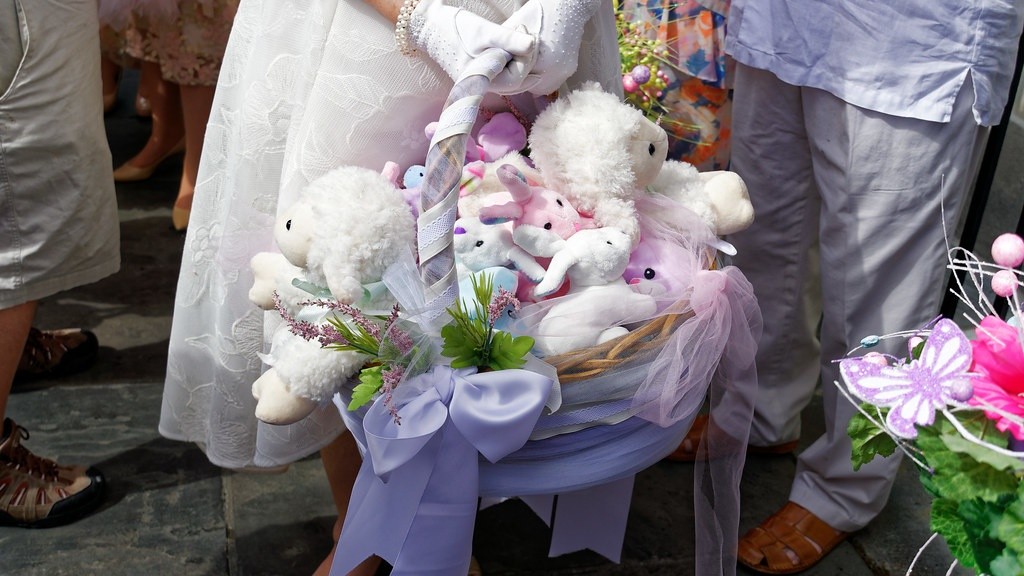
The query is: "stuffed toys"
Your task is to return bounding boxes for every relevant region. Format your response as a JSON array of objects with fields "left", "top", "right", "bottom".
[
  {"left": 380, "top": 76, "right": 758, "bottom": 357},
  {"left": 250, "top": 165, "right": 418, "bottom": 425}
]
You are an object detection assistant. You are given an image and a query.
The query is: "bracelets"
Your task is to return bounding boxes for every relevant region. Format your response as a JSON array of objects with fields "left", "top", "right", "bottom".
[{"left": 395, "top": 0, "right": 418, "bottom": 56}]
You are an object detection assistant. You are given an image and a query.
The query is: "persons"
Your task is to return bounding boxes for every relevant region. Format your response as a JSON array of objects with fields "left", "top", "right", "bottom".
[
  {"left": 0, "top": 0, "right": 241, "bottom": 529},
  {"left": 158, "top": 0, "right": 624, "bottom": 576},
  {"left": 618, "top": 0, "right": 1024, "bottom": 576}
]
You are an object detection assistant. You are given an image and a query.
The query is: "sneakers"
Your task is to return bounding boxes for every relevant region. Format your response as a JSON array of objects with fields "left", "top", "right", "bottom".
[
  {"left": 10, "top": 326, "right": 100, "bottom": 381},
  {"left": 0, "top": 414, "right": 108, "bottom": 529}
]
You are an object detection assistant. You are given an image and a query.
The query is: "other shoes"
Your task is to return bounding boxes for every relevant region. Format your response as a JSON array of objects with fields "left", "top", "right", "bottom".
[
  {"left": 135, "top": 90, "right": 152, "bottom": 118},
  {"left": 104, "top": 81, "right": 121, "bottom": 113}
]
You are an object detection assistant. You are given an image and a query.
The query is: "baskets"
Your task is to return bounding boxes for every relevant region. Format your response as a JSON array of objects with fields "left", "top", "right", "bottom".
[{"left": 332, "top": 234, "right": 728, "bottom": 497}]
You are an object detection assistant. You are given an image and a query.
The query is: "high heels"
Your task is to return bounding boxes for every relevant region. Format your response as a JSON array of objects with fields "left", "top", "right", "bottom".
[
  {"left": 114, "top": 134, "right": 187, "bottom": 180},
  {"left": 172, "top": 177, "right": 189, "bottom": 233}
]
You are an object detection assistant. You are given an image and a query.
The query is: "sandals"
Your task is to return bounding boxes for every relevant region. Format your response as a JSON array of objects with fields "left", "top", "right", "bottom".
[
  {"left": 730, "top": 499, "right": 850, "bottom": 576},
  {"left": 660, "top": 416, "right": 802, "bottom": 462}
]
